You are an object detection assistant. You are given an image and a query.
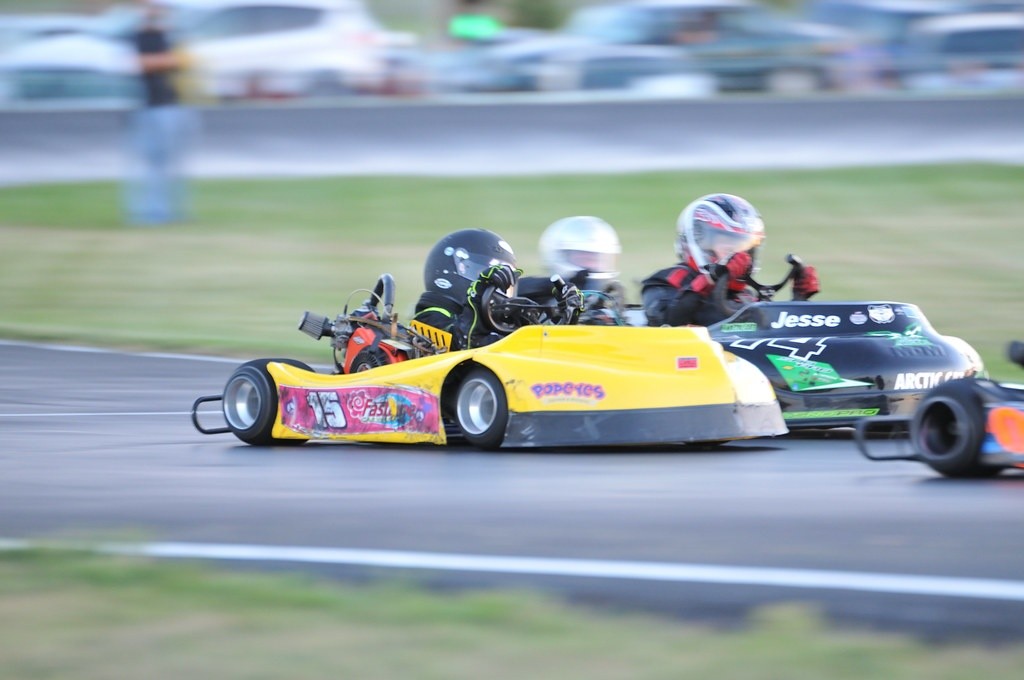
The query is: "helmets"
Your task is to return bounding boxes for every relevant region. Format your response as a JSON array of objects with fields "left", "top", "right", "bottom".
[
  {"left": 423, "top": 227, "right": 518, "bottom": 308},
  {"left": 536, "top": 213, "right": 622, "bottom": 290},
  {"left": 675, "top": 192, "right": 765, "bottom": 274}
]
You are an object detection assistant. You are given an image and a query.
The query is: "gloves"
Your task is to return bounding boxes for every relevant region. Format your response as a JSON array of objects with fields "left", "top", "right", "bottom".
[
  {"left": 467, "top": 263, "right": 523, "bottom": 303},
  {"left": 704, "top": 250, "right": 751, "bottom": 283},
  {"left": 782, "top": 268, "right": 819, "bottom": 301},
  {"left": 551, "top": 282, "right": 585, "bottom": 325}
]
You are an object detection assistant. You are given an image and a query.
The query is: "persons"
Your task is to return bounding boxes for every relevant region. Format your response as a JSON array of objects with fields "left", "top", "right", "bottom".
[
  {"left": 642, "top": 192, "right": 821, "bottom": 328},
  {"left": 534, "top": 210, "right": 647, "bottom": 325},
  {"left": 593, "top": 1, "right": 1016, "bottom": 97},
  {"left": 408, "top": 227, "right": 547, "bottom": 352},
  {"left": 448, "top": 0, "right": 532, "bottom": 92},
  {"left": 121, "top": 1, "right": 187, "bottom": 108}
]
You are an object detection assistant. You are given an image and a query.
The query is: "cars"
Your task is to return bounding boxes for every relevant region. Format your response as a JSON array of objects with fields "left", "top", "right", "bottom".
[{"left": 0, "top": 0, "right": 1023, "bottom": 107}]
